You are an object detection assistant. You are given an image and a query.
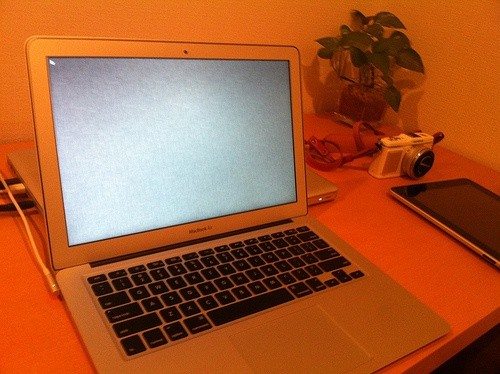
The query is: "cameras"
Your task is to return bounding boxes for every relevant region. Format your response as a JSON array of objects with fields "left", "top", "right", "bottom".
[{"left": 369, "top": 134, "right": 437, "bottom": 179}]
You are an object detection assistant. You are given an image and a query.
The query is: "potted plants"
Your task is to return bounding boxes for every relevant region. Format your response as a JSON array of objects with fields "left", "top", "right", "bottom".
[{"left": 313, "top": 9, "right": 425, "bottom": 130}]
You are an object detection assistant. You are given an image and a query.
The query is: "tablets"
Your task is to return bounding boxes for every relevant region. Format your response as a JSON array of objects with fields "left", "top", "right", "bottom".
[{"left": 387, "top": 176, "right": 500, "bottom": 269}]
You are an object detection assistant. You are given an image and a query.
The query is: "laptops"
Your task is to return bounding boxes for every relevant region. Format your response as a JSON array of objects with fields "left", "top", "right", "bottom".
[
  {"left": 27, "top": 37, "right": 449, "bottom": 374},
  {"left": 10, "top": 149, "right": 340, "bottom": 216}
]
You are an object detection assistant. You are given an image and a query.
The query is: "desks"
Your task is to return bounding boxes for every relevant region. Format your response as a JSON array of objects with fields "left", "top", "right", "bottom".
[{"left": 1, "top": 107, "right": 498, "bottom": 374}]
